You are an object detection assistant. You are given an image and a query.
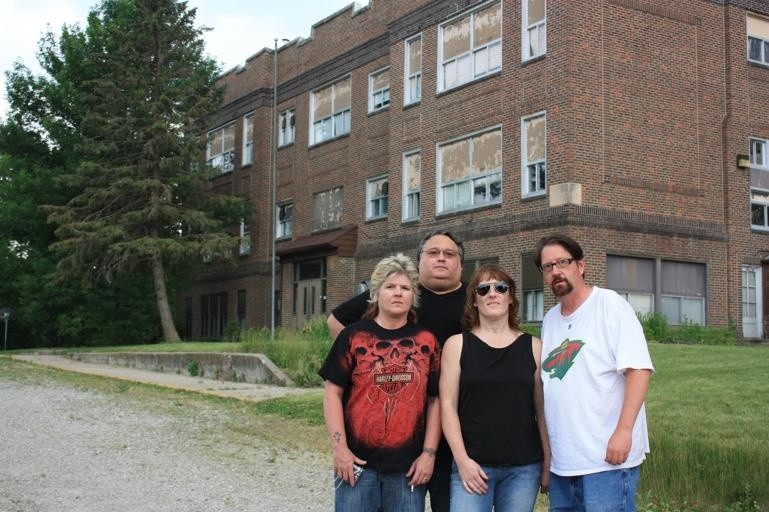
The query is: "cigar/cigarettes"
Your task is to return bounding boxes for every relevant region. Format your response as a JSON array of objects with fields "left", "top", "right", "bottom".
[{"left": 411, "top": 485, "right": 414, "bottom": 493}]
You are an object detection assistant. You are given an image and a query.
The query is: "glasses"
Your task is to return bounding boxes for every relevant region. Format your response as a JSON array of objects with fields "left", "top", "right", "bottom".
[
  {"left": 421, "top": 248, "right": 459, "bottom": 259},
  {"left": 475, "top": 282, "right": 509, "bottom": 296},
  {"left": 540, "top": 257, "right": 575, "bottom": 273}
]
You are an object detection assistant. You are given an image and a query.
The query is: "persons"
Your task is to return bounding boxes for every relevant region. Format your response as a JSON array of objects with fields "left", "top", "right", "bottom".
[
  {"left": 318, "top": 252, "right": 442, "bottom": 512},
  {"left": 438, "top": 263, "right": 551, "bottom": 512},
  {"left": 533, "top": 234, "right": 655, "bottom": 512},
  {"left": 326, "top": 229, "right": 471, "bottom": 512}
]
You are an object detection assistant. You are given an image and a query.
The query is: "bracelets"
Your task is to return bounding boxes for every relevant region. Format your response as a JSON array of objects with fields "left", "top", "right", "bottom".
[{"left": 423, "top": 447, "right": 437, "bottom": 455}]
